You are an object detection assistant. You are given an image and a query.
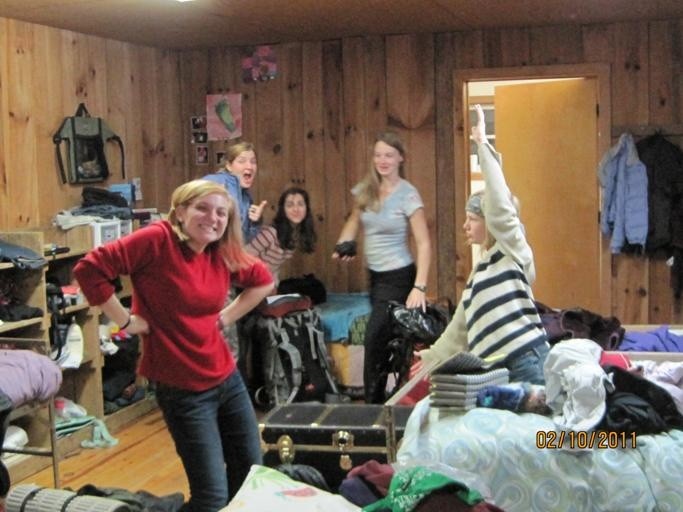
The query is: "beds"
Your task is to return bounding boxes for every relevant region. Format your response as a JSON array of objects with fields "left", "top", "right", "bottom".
[
  {"left": 311, "top": 288, "right": 398, "bottom": 393},
  {"left": 413, "top": 323, "right": 683, "bottom": 511}
]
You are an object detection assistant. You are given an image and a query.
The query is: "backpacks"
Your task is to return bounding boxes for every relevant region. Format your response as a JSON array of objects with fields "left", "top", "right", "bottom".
[{"left": 252, "top": 307, "right": 342, "bottom": 407}]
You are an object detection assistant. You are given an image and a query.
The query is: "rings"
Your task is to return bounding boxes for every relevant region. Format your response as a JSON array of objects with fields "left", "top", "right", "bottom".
[{"left": 251, "top": 208, "right": 257, "bottom": 213}]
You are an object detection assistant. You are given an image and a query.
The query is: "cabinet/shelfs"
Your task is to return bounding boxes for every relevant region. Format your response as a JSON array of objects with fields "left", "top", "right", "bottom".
[{"left": 0, "top": 211, "right": 166, "bottom": 489}]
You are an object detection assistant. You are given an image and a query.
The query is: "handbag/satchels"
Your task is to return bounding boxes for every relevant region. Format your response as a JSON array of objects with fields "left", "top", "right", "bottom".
[{"left": 392, "top": 303, "right": 447, "bottom": 344}]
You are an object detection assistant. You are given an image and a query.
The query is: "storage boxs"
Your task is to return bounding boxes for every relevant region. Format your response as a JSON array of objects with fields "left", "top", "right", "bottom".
[{"left": 258, "top": 402, "right": 421, "bottom": 490}]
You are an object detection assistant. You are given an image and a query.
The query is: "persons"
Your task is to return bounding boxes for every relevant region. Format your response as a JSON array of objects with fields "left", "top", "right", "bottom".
[
  {"left": 71, "top": 178, "right": 276, "bottom": 510},
  {"left": 328, "top": 131, "right": 432, "bottom": 406},
  {"left": 204, "top": 140, "right": 268, "bottom": 365},
  {"left": 237, "top": 186, "right": 318, "bottom": 408},
  {"left": 406, "top": 99, "right": 553, "bottom": 386}
]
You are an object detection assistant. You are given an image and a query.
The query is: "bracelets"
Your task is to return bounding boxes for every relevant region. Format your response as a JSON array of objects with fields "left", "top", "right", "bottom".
[
  {"left": 412, "top": 283, "right": 428, "bottom": 292},
  {"left": 118, "top": 315, "right": 131, "bottom": 331},
  {"left": 216, "top": 312, "right": 225, "bottom": 333}
]
